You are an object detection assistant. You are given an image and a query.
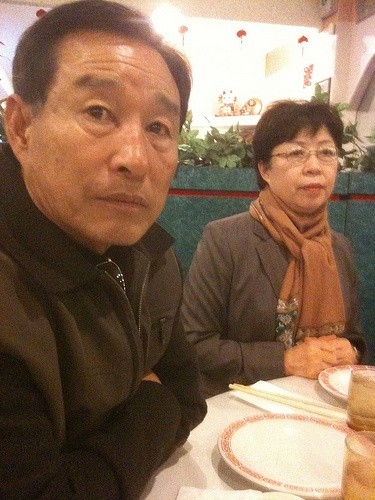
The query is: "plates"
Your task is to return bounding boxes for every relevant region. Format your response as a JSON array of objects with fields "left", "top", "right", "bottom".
[
  {"left": 218, "top": 413, "right": 375, "bottom": 500},
  {"left": 319, "top": 365, "right": 375, "bottom": 401}
]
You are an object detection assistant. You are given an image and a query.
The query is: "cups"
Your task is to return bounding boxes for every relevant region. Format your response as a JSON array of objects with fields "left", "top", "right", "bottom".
[
  {"left": 348, "top": 369, "right": 375, "bottom": 430},
  {"left": 343, "top": 432, "right": 375, "bottom": 500}
]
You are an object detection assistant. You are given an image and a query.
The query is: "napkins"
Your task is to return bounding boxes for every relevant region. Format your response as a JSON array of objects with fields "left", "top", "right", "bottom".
[{"left": 230, "top": 380, "right": 310, "bottom": 414}]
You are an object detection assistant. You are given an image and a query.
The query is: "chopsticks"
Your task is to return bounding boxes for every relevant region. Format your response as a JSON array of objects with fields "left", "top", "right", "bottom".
[{"left": 229, "top": 383, "right": 348, "bottom": 420}]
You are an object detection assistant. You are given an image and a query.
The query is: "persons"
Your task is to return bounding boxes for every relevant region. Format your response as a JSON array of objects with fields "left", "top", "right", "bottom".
[
  {"left": 0, "top": 0, "right": 203, "bottom": 500},
  {"left": 180, "top": 95, "right": 371, "bottom": 402}
]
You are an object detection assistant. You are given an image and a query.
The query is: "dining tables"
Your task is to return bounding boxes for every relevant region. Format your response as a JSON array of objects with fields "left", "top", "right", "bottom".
[{"left": 138, "top": 375, "right": 375, "bottom": 500}]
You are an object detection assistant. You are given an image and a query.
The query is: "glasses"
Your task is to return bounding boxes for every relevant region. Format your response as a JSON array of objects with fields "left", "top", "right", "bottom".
[
  {"left": 95, "top": 257, "right": 127, "bottom": 296},
  {"left": 270, "top": 145, "right": 339, "bottom": 162}
]
